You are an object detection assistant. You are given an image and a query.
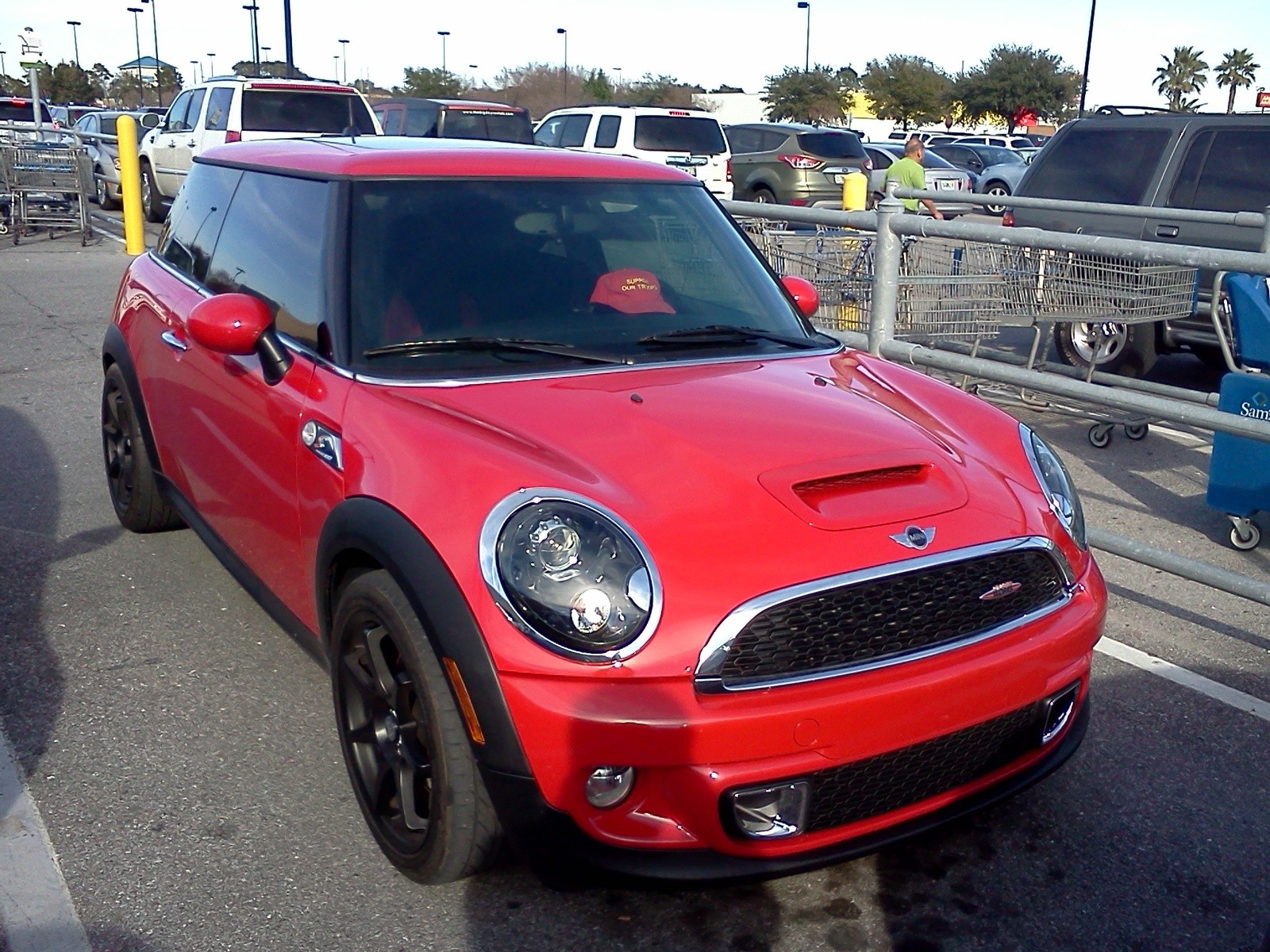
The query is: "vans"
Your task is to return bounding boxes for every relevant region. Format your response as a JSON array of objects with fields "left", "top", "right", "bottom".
[{"left": 519, "top": 108, "right": 733, "bottom": 219}]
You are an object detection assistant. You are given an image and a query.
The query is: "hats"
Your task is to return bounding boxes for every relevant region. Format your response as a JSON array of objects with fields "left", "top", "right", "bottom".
[{"left": 590, "top": 269, "right": 676, "bottom": 315}]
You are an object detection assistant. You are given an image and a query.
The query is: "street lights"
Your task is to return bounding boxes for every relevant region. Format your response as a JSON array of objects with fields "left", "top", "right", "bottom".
[
  {"left": 555, "top": 28, "right": 577, "bottom": 109},
  {"left": 0, "top": 0, "right": 452, "bottom": 100},
  {"left": 796, "top": 2, "right": 813, "bottom": 72}
]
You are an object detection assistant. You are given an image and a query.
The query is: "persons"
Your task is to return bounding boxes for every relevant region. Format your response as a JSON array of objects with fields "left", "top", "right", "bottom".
[{"left": 881, "top": 138, "right": 942, "bottom": 242}]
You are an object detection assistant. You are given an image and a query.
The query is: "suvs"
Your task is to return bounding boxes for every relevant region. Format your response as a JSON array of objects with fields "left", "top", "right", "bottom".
[
  {"left": 889, "top": 124, "right": 1036, "bottom": 163},
  {"left": 0, "top": 95, "right": 56, "bottom": 149},
  {"left": 714, "top": 123, "right": 873, "bottom": 206},
  {"left": 139, "top": 76, "right": 376, "bottom": 224},
  {"left": 1002, "top": 106, "right": 1270, "bottom": 375},
  {"left": 371, "top": 99, "right": 535, "bottom": 150}
]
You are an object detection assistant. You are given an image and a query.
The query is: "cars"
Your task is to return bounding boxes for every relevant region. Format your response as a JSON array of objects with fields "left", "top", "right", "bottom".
[
  {"left": 46, "top": 106, "right": 172, "bottom": 212},
  {"left": 99, "top": 139, "right": 1108, "bottom": 895},
  {"left": 860, "top": 144, "right": 1045, "bottom": 216}
]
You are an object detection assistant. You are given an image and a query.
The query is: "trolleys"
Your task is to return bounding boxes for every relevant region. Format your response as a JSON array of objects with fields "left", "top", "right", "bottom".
[
  {"left": 751, "top": 206, "right": 1204, "bottom": 446},
  {"left": 1207, "top": 270, "right": 1269, "bottom": 555},
  {"left": 1, "top": 130, "right": 95, "bottom": 245}
]
